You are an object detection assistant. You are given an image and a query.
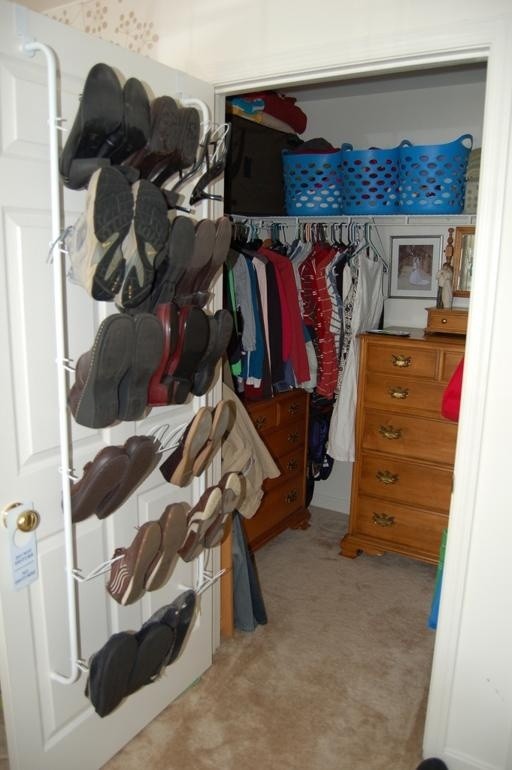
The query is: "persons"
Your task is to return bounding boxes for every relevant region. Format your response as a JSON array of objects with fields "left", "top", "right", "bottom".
[{"left": 437, "top": 263, "right": 453, "bottom": 310}]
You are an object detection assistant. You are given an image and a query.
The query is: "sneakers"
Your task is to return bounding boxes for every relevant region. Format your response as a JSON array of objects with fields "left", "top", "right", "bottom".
[
  {"left": 205, "top": 472, "right": 241, "bottom": 548},
  {"left": 107, "top": 521, "right": 162, "bottom": 606},
  {"left": 177, "top": 486, "right": 222, "bottom": 562},
  {"left": 144, "top": 503, "right": 187, "bottom": 591}
]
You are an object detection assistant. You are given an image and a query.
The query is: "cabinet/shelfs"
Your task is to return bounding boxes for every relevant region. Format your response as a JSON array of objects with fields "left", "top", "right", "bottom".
[
  {"left": 337, "top": 326, "right": 469, "bottom": 566},
  {"left": 225, "top": 384, "right": 312, "bottom": 552}
]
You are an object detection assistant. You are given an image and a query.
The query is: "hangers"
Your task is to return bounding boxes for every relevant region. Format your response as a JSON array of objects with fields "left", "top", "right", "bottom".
[{"left": 231, "top": 218, "right": 388, "bottom": 276}]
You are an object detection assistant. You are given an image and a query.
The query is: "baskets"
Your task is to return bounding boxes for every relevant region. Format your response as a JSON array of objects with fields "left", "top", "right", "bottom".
[{"left": 281, "top": 133, "right": 473, "bottom": 214}]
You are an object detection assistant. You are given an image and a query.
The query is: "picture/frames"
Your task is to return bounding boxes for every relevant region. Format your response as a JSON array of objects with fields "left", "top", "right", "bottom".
[
  {"left": 450, "top": 226, "right": 476, "bottom": 297},
  {"left": 387, "top": 235, "right": 444, "bottom": 300}
]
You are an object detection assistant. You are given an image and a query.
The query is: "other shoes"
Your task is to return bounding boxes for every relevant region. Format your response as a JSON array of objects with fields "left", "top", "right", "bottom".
[
  {"left": 161, "top": 593, "right": 196, "bottom": 666},
  {"left": 130, "top": 622, "right": 174, "bottom": 697},
  {"left": 191, "top": 399, "right": 237, "bottom": 478},
  {"left": 85, "top": 632, "right": 139, "bottom": 717},
  {"left": 160, "top": 399, "right": 213, "bottom": 487}
]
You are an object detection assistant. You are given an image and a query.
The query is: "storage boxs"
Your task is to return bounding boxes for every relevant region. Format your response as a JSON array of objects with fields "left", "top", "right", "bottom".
[{"left": 423, "top": 308, "right": 468, "bottom": 337}]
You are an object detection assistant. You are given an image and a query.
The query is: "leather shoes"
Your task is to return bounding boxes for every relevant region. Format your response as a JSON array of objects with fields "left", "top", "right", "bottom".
[
  {"left": 59, "top": 63, "right": 233, "bottom": 428},
  {"left": 71, "top": 435, "right": 129, "bottom": 523},
  {"left": 95, "top": 435, "right": 156, "bottom": 519}
]
